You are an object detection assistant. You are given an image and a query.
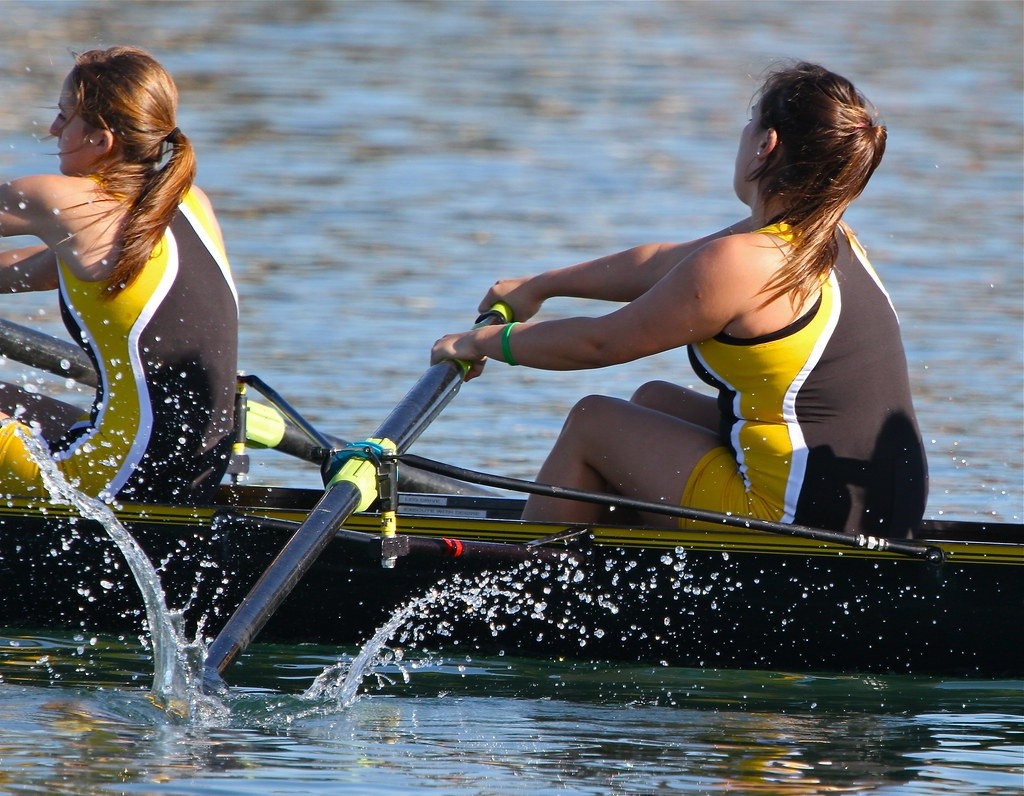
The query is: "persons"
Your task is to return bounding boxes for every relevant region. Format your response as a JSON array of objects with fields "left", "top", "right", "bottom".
[
  {"left": 429, "top": 56, "right": 930, "bottom": 538},
  {"left": 0, "top": 45, "right": 239, "bottom": 504}
]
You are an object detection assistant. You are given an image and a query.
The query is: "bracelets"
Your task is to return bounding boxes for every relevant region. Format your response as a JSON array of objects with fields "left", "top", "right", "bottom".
[{"left": 500, "top": 322, "right": 521, "bottom": 367}]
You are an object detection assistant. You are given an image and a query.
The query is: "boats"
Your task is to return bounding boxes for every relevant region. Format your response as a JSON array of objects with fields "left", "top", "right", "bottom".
[{"left": 1, "top": 482, "right": 1024, "bottom": 683}]
